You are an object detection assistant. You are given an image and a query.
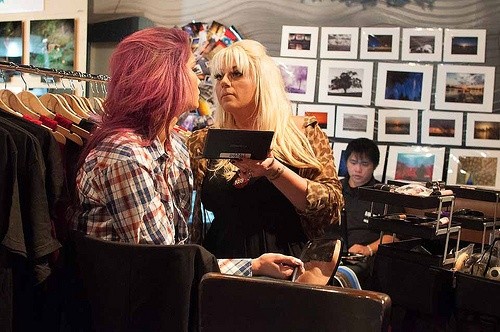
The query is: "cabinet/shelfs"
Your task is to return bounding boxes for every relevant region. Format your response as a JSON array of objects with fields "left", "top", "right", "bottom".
[{"left": 355, "top": 186, "right": 500, "bottom": 271}]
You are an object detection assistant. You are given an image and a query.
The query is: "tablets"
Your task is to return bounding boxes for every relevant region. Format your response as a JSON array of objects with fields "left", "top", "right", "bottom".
[{"left": 202, "top": 128, "right": 275, "bottom": 160}]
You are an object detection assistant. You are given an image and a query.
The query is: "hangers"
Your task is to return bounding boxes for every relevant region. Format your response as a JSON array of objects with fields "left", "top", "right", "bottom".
[{"left": 0, "top": 61, "right": 112, "bottom": 146}]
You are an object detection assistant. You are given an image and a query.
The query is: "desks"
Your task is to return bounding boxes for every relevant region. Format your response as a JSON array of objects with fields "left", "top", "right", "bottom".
[{"left": 366, "top": 254, "right": 500, "bottom": 332}]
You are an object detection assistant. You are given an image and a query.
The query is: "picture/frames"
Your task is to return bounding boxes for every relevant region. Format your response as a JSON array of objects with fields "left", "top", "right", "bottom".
[{"left": 271, "top": 25, "right": 500, "bottom": 193}]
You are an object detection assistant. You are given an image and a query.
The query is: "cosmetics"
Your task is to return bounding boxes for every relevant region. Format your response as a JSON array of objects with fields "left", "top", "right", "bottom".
[{"left": 195, "top": 128, "right": 276, "bottom": 160}]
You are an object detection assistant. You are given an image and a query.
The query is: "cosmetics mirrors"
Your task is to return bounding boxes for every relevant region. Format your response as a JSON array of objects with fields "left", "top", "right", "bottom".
[{"left": 292, "top": 236, "right": 344, "bottom": 284}]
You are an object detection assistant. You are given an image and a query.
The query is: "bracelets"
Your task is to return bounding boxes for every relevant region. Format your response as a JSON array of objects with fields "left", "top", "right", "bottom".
[
  {"left": 367, "top": 244, "right": 373, "bottom": 258},
  {"left": 270, "top": 164, "right": 285, "bottom": 180}
]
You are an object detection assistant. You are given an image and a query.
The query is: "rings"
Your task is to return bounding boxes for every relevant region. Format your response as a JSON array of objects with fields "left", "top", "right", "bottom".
[{"left": 248, "top": 169, "right": 253, "bottom": 174}]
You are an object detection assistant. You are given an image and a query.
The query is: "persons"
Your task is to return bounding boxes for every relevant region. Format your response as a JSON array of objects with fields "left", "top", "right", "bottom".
[
  {"left": 188, "top": 39, "right": 344, "bottom": 279},
  {"left": 333, "top": 137, "right": 404, "bottom": 281},
  {"left": 73, "top": 23, "right": 303, "bottom": 281}
]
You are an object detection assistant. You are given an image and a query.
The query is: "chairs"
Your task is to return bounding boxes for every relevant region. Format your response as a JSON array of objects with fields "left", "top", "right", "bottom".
[
  {"left": 60, "top": 230, "right": 220, "bottom": 332},
  {"left": 198, "top": 272, "right": 392, "bottom": 332}
]
